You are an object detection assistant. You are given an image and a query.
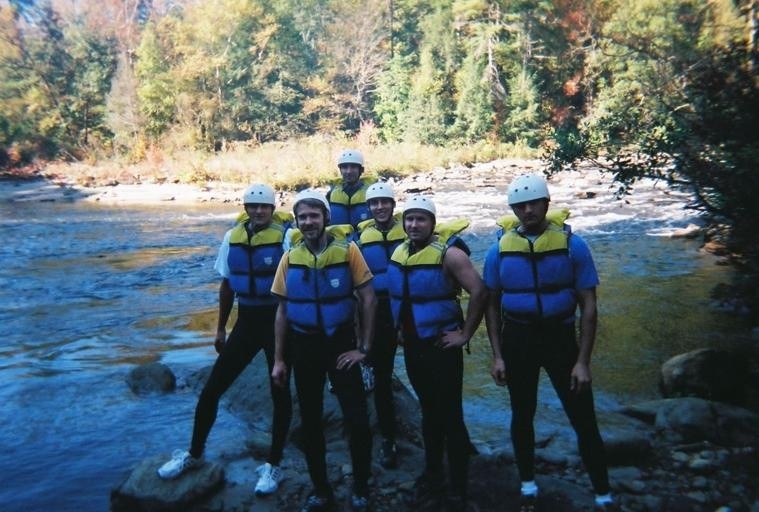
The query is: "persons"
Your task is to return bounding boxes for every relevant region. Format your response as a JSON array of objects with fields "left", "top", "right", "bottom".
[
  {"left": 324, "top": 149, "right": 374, "bottom": 226},
  {"left": 158, "top": 183, "right": 291, "bottom": 495},
  {"left": 387, "top": 197, "right": 489, "bottom": 504},
  {"left": 482, "top": 174, "right": 615, "bottom": 506},
  {"left": 270, "top": 190, "right": 380, "bottom": 512},
  {"left": 355, "top": 182, "right": 408, "bottom": 468}
]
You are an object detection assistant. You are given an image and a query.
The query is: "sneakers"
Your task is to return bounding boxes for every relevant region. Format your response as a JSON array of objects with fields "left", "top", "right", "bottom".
[
  {"left": 404, "top": 474, "right": 439, "bottom": 512},
  {"left": 348, "top": 485, "right": 373, "bottom": 512},
  {"left": 254, "top": 459, "right": 285, "bottom": 498},
  {"left": 378, "top": 436, "right": 398, "bottom": 468},
  {"left": 518, "top": 491, "right": 539, "bottom": 512},
  {"left": 435, "top": 490, "right": 469, "bottom": 512},
  {"left": 594, "top": 494, "right": 625, "bottom": 512},
  {"left": 156, "top": 449, "right": 207, "bottom": 480},
  {"left": 360, "top": 361, "right": 377, "bottom": 393},
  {"left": 302, "top": 492, "right": 337, "bottom": 512}
]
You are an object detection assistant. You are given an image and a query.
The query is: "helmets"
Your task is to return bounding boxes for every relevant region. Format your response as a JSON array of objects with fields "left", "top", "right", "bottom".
[
  {"left": 508, "top": 174, "right": 550, "bottom": 205},
  {"left": 242, "top": 183, "right": 275, "bottom": 209},
  {"left": 366, "top": 182, "right": 396, "bottom": 209},
  {"left": 402, "top": 196, "right": 437, "bottom": 226},
  {"left": 290, "top": 189, "right": 331, "bottom": 225},
  {"left": 337, "top": 147, "right": 365, "bottom": 172}
]
